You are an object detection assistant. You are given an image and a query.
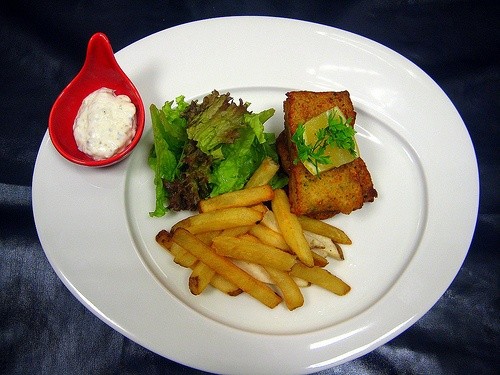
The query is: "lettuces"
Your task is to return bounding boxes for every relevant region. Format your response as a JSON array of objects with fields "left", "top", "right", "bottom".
[{"left": 145, "top": 91, "right": 289, "bottom": 218}]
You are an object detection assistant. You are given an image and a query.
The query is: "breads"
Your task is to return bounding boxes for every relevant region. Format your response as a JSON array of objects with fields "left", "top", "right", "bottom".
[{"left": 277, "top": 90, "right": 378, "bottom": 221}]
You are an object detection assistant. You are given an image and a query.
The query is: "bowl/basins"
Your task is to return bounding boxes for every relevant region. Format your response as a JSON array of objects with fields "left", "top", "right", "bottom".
[{"left": 48, "top": 31, "right": 147, "bottom": 169}]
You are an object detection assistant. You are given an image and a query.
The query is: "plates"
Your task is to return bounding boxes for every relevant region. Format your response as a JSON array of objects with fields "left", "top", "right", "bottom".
[{"left": 32, "top": 16, "right": 480, "bottom": 375}]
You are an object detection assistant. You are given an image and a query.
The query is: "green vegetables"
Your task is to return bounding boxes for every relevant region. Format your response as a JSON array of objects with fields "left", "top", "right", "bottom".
[{"left": 291, "top": 108, "right": 357, "bottom": 179}]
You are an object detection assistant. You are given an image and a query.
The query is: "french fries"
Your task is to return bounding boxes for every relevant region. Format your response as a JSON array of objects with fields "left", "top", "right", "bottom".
[{"left": 154, "top": 158, "right": 353, "bottom": 312}]
